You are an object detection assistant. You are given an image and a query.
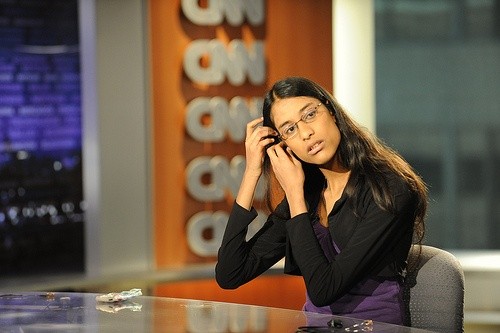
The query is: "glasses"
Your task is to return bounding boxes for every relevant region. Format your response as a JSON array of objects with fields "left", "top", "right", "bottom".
[{"left": 278, "top": 102, "right": 323, "bottom": 142}]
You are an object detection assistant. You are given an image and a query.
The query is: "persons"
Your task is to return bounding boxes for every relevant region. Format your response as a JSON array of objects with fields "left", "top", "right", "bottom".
[{"left": 215, "top": 78, "right": 426, "bottom": 326}]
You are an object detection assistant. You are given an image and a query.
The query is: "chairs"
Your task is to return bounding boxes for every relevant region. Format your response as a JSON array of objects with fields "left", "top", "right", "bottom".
[{"left": 405, "top": 244, "right": 465, "bottom": 333}]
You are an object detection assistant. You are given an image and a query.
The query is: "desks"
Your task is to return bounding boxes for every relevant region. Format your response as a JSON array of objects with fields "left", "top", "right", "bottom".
[{"left": 0, "top": 291, "right": 435, "bottom": 333}]
